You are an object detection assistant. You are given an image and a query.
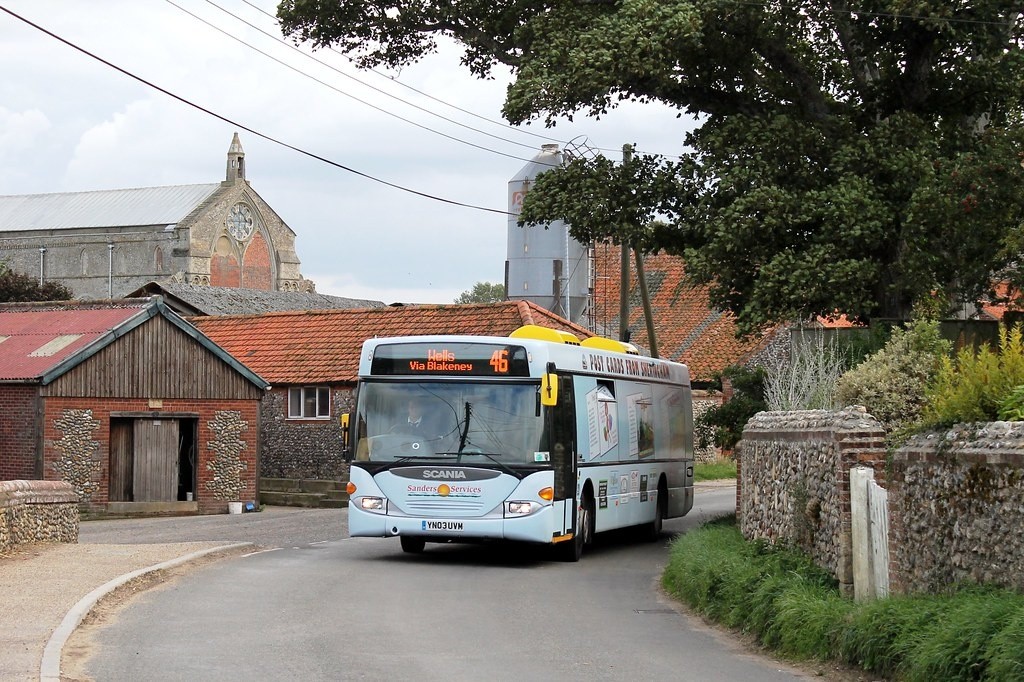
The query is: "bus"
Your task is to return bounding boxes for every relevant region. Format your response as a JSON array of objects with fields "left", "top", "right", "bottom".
[{"left": 340, "top": 325, "right": 696, "bottom": 562}]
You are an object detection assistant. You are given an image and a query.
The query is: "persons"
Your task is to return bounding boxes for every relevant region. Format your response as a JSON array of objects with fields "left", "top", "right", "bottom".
[{"left": 392, "top": 398, "right": 430, "bottom": 438}]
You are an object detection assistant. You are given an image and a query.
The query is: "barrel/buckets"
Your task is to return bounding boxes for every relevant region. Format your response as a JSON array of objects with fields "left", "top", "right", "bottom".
[
  {"left": 228, "top": 501, "right": 243, "bottom": 515},
  {"left": 186, "top": 492, "right": 193, "bottom": 501}
]
